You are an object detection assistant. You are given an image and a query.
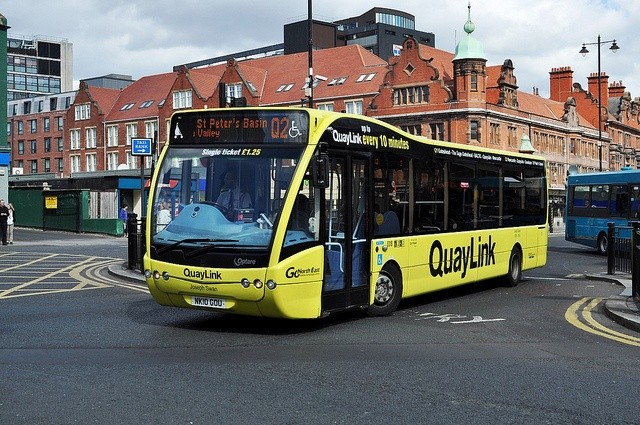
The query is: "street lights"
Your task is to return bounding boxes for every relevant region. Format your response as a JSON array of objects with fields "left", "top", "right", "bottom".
[{"left": 578, "top": 34, "right": 619, "bottom": 172}]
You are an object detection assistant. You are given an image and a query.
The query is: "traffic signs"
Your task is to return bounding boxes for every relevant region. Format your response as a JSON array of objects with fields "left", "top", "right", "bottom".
[{"left": 130, "top": 137, "right": 152, "bottom": 157}]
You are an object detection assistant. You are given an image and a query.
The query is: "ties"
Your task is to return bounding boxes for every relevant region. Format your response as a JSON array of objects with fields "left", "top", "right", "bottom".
[{"left": 228, "top": 190, "right": 233, "bottom": 211}]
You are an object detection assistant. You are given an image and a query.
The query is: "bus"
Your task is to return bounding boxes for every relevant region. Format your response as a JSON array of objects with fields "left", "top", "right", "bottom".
[
  {"left": 143, "top": 107, "right": 549, "bottom": 328},
  {"left": 563, "top": 167, "right": 640, "bottom": 253}
]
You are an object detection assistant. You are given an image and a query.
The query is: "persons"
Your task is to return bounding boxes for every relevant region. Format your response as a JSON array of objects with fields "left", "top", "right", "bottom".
[
  {"left": 6, "top": 204, "right": 15, "bottom": 244},
  {"left": 0, "top": 199, "right": 9, "bottom": 245},
  {"left": 214, "top": 172, "right": 253, "bottom": 222},
  {"left": 120, "top": 204, "right": 128, "bottom": 238}
]
